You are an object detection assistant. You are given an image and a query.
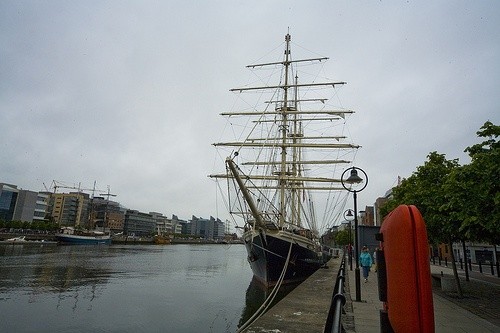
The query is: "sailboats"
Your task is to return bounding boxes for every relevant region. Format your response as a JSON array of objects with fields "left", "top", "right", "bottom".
[{"left": 207, "top": 26, "right": 363, "bottom": 289}]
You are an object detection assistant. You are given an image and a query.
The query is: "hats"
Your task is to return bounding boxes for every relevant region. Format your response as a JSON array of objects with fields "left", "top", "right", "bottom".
[{"left": 361, "top": 246, "right": 369, "bottom": 251}]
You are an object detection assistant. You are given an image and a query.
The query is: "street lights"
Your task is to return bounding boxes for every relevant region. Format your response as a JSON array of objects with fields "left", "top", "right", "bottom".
[
  {"left": 341, "top": 165, "right": 369, "bottom": 303},
  {"left": 341, "top": 208, "right": 355, "bottom": 271}
]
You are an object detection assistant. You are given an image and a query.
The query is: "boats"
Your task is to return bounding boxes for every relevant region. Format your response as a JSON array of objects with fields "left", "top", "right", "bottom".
[
  {"left": 54, "top": 226, "right": 111, "bottom": 245},
  {"left": 0, "top": 235, "right": 32, "bottom": 244}
]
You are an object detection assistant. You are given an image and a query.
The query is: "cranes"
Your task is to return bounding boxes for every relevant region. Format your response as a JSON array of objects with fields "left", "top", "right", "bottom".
[{"left": 42, "top": 179, "right": 117, "bottom": 200}]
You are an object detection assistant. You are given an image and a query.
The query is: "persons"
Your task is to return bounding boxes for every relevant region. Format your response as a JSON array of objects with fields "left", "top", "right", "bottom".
[
  {"left": 359, "top": 247, "right": 372, "bottom": 283},
  {"left": 351, "top": 243, "right": 356, "bottom": 257},
  {"left": 372, "top": 248, "right": 378, "bottom": 272}
]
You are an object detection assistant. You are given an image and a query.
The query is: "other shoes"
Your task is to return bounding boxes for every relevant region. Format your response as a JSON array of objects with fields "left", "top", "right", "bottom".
[{"left": 364, "top": 279, "right": 367, "bottom": 282}]
